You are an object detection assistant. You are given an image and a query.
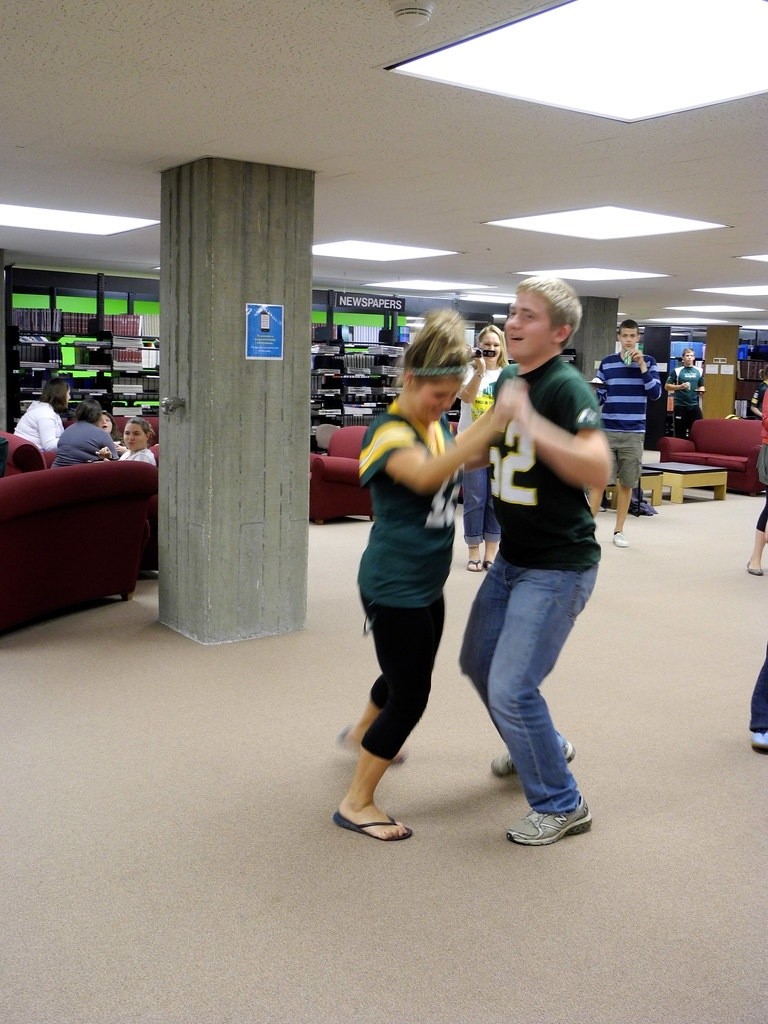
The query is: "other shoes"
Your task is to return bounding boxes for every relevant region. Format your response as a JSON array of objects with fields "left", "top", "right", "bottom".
[{"left": 745, "top": 561, "right": 763, "bottom": 576}]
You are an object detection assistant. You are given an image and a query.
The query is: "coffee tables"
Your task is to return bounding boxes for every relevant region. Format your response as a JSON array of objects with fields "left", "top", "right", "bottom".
[
  {"left": 642, "top": 462, "right": 727, "bottom": 503},
  {"left": 606, "top": 469, "right": 663, "bottom": 506}
]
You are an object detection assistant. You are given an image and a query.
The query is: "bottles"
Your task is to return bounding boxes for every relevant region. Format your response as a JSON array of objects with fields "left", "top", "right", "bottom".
[{"left": 624, "top": 344, "right": 632, "bottom": 366}]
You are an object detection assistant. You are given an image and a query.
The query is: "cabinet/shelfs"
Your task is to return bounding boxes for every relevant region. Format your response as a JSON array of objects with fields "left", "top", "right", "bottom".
[
  {"left": 5, "top": 318, "right": 160, "bottom": 434},
  {"left": 311, "top": 339, "right": 411, "bottom": 428}
]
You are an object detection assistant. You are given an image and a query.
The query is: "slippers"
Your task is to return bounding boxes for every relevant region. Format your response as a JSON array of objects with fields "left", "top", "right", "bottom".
[
  {"left": 485, "top": 560, "right": 496, "bottom": 572},
  {"left": 338, "top": 731, "right": 410, "bottom": 766},
  {"left": 332, "top": 808, "right": 413, "bottom": 840},
  {"left": 467, "top": 561, "right": 483, "bottom": 573}
]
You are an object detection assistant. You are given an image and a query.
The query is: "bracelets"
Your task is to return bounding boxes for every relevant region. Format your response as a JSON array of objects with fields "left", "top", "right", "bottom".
[{"left": 476, "top": 373, "right": 484, "bottom": 377}]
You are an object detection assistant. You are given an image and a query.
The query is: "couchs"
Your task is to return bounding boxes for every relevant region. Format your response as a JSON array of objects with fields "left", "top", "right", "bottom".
[
  {"left": 658, "top": 418, "right": 766, "bottom": 496},
  {"left": 0, "top": 417, "right": 159, "bottom": 634},
  {"left": 309, "top": 426, "right": 375, "bottom": 525}
]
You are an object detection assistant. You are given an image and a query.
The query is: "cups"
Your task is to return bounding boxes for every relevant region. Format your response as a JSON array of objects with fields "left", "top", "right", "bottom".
[{"left": 686, "top": 382, "right": 690, "bottom": 391}]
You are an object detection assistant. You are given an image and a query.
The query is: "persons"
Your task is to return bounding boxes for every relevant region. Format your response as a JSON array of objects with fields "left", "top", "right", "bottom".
[
  {"left": 51, "top": 400, "right": 118, "bottom": 467},
  {"left": 665, "top": 348, "right": 705, "bottom": 438},
  {"left": 589, "top": 320, "right": 661, "bottom": 546},
  {"left": 746, "top": 388, "right": 768, "bottom": 576},
  {"left": 95, "top": 417, "right": 156, "bottom": 466},
  {"left": 99, "top": 412, "right": 128, "bottom": 456},
  {"left": 750, "top": 642, "right": 768, "bottom": 749},
  {"left": 459, "top": 277, "right": 610, "bottom": 845},
  {"left": 750, "top": 364, "right": 768, "bottom": 420},
  {"left": 458, "top": 325, "right": 509, "bottom": 572},
  {"left": 334, "top": 309, "right": 514, "bottom": 842},
  {"left": 13, "top": 378, "right": 71, "bottom": 452}
]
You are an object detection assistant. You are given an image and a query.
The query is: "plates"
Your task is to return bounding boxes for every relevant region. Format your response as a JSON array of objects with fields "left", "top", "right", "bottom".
[{"left": 694, "top": 390, "right": 707, "bottom": 393}]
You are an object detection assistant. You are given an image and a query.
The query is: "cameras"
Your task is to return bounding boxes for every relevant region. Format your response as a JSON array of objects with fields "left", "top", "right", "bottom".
[{"left": 475, "top": 349, "right": 496, "bottom": 357}]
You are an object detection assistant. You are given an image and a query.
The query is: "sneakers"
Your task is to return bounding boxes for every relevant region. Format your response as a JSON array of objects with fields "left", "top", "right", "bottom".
[
  {"left": 613, "top": 533, "right": 630, "bottom": 548},
  {"left": 491, "top": 744, "right": 574, "bottom": 776},
  {"left": 505, "top": 795, "right": 592, "bottom": 846}
]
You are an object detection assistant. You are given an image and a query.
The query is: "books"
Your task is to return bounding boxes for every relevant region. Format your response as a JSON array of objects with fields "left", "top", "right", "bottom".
[
  {"left": 735, "top": 360, "right": 768, "bottom": 418},
  {"left": 12, "top": 308, "right": 160, "bottom": 399},
  {"left": 312, "top": 323, "right": 375, "bottom": 426}
]
